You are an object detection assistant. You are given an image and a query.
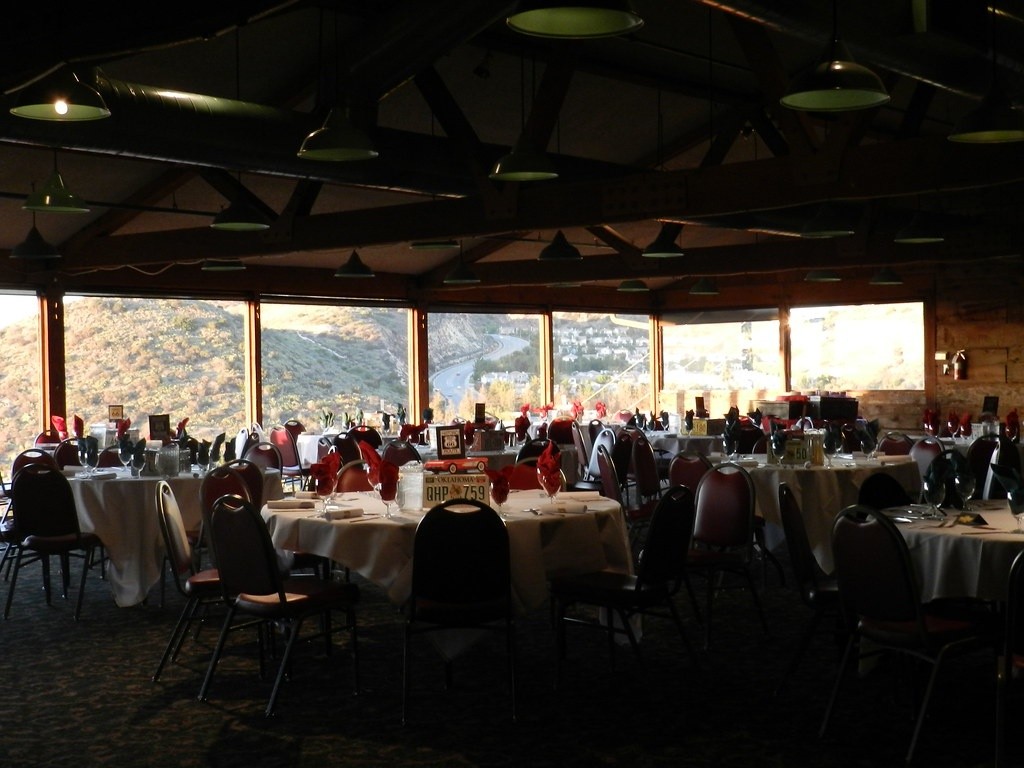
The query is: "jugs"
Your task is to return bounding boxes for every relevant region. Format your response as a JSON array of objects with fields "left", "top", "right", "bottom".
[
  {"left": 396, "top": 466, "right": 424, "bottom": 510},
  {"left": 154, "top": 447, "right": 179, "bottom": 478}
]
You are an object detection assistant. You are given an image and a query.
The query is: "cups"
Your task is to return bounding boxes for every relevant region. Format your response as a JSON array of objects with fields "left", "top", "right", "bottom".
[
  {"left": 509, "top": 434, "right": 516, "bottom": 448},
  {"left": 116, "top": 432, "right": 183, "bottom": 444},
  {"left": 58, "top": 430, "right": 82, "bottom": 442},
  {"left": 196, "top": 452, "right": 221, "bottom": 471}
]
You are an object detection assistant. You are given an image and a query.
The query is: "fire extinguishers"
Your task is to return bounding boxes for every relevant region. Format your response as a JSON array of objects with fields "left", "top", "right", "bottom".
[{"left": 952, "top": 349, "right": 965, "bottom": 379}]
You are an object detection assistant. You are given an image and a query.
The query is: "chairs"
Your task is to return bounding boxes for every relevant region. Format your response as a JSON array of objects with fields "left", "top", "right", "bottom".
[{"left": 0, "top": 407, "right": 1024, "bottom": 768}]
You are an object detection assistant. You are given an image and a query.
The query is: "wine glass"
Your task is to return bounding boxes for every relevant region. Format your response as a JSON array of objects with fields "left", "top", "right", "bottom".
[
  {"left": 635, "top": 415, "right": 668, "bottom": 436},
  {"left": 860, "top": 440, "right": 875, "bottom": 466},
  {"left": 314, "top": 479, "right": 397, "bottom": 518},
  {"left": 1006, "top": 491, "right": 1024, "bottom": 534},
  {"left": 489, "top": 468, "right": 564, "bottom": 520},
  {"left": 685, "top": 419, "right": 692, "bottom": 437},
  {"left": 78, "top": 448, "right": 146, "bottom": 479},
  {"left": 923, "top": 475, "right": 975, "bottom": 525},
  {"left": 400, "top": 429, "right": 547, "bottom": 455},
  {"left": 722, "top": 440, "right": 836, "bottom": 467},
  {"left": 924, "top": 421, "right": 1018, "bottom": 441}
]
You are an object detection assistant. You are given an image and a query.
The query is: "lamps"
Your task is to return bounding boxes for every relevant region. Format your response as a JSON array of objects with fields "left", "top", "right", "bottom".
[{"left": 0, "top": 0, "right": 1024, "bottom": 296}]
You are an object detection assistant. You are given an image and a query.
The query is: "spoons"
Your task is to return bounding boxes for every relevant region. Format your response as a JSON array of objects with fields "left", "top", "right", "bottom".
[{"left": 529, "top": 508, "right": 562, "bottom": 516}]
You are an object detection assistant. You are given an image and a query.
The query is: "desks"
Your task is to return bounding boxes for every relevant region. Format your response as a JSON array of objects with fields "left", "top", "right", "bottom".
[
  {"left": 260, "top": 485, "right": 635, "bottom": 696},
  {"left": 749, "top": 399, "right": 810, "bottom": 422},
  {"left": 906, "top": 435, "right": 1024, "bottom": 461},
  {"left": 370, "top": 446, "right": 577, "bottom": 491},
  {"left": 641, "top": 431, "right": 731, "bottom": 459},
  {"left": 38, "top": 465, "right": 285, "bottom": 612},
  {"left": 685, "top": 453, "right": 923, "bottom": 577},
  {"left": 879, "top": 499, "right": 1024, "bottom": 616}
]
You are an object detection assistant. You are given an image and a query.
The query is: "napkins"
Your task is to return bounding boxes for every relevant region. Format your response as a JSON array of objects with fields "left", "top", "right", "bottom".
[
  {"left": 515, "top": 415, "right": 529, "bottom": 441},
  {"left": 50, "top": 415, "right": 69, "bottom": 441},
  {"left": 356, "top": 439, "right": 383, "bottom": 486},
  {"left": 117, "top": 418, "right": 132, "bottom": 440},
  {"left": 309, "top": 450, "right": 341, "bottom": 496},
  {"left": 536, "top": 440, "right": 563, "bottom": 496}
]
